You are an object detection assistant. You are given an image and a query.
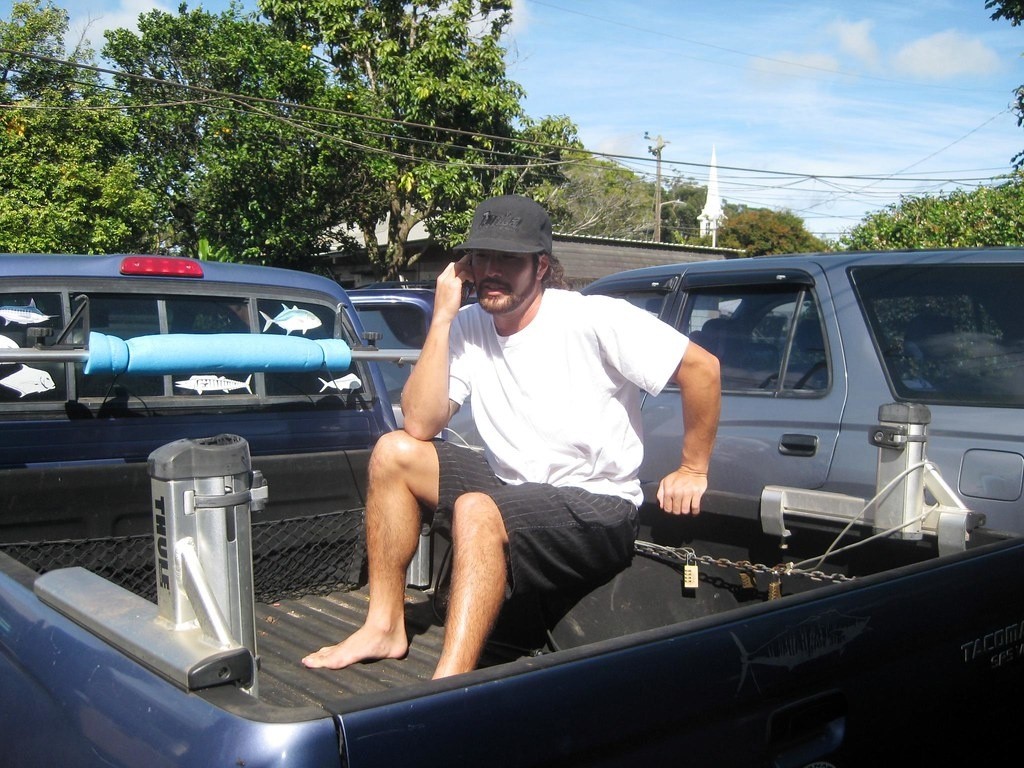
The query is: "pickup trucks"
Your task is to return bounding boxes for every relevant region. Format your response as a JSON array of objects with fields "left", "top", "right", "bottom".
[{"left": 0, "top": 253, "right": 1024, "bottom": 768}]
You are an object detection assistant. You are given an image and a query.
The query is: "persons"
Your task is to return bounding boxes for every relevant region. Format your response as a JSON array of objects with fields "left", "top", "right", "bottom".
[{"left": 302, "top": 196, "right": 721, "bottom": 682}]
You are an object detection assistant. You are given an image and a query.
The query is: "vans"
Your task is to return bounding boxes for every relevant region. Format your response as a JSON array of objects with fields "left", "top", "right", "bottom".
[{"left": 557, "top": 249, "right": 1024, "bottom": 543}]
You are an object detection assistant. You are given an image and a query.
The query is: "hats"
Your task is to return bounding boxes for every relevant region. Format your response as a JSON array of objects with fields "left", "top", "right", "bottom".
[{"left": 453, "top": 194, "right": 553, "bottom": 257}]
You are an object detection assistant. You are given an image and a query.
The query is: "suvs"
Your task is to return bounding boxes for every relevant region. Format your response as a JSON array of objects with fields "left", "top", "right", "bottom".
[{"left": 344, "top": 280, "right": 482, "bottom": 441}]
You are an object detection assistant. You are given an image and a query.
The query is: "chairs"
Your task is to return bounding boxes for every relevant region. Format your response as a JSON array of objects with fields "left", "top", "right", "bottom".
[
  {"left": 702, "top": 319, "right": 745, "bottom": 370},
  {"left": 787, "top": 318, "right": 828, "bottom": 389},
  {"left": 899, "top": 316, "right": 956, "bottom": 384},
  {"left": 171, "top": 305, "right": 244, "bottom": 399}
]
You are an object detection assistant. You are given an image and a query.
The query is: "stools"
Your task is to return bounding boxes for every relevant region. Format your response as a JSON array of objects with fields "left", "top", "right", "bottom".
[{"left": 423, "top": 516, "right": 568, "bottom": 657}]
